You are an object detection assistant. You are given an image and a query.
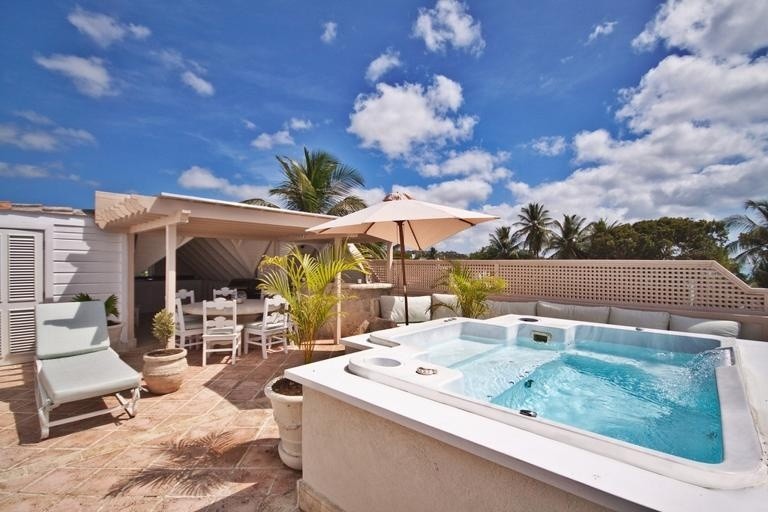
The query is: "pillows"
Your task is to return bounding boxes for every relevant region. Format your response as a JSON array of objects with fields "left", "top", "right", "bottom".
[
  {"left": 378, "top": 293, "right": 431, "bottom": 324},
  {"left": 434, "top": 293, "right": 467, "bottom": 319},
  {"left": 536, "top": 301, "right": 609, "bottom": 327},
  {"left": 668, "top": 313, "right": 742, "bottom": 340},
  {"left": 467, "top": 295, "right": 536, "bottom": 320},
  {"left": 610, "top": 305, "right": 670, "bottom": 331}
]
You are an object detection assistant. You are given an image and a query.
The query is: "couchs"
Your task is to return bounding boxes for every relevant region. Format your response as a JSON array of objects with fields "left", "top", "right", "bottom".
[{"left": 366, "top": 291, "right": 767, "bottom": 351}]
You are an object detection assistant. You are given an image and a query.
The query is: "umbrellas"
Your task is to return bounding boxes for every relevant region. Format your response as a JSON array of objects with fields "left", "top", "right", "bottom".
[{"left": 305, "top": 191, "right": 500, "bottom": 325}]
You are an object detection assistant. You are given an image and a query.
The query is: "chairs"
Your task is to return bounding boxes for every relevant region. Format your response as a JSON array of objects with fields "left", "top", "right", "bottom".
[
  {"left": 172, "top": 283, "right": 291, "bottom": 367},
  {"left": 32, "top": 300, "right": 145, "bottom": 439}
]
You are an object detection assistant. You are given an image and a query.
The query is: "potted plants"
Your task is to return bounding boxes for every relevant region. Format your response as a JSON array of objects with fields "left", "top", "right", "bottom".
[
  {"left": 140, "top": 309, "right": 189, "bottom": 395},
  {"left": 254, "top": 234, "right": 372, "bottom": 472},
  {"left": 72, "top": 292, "right": 124, "bottom": 352}
]
